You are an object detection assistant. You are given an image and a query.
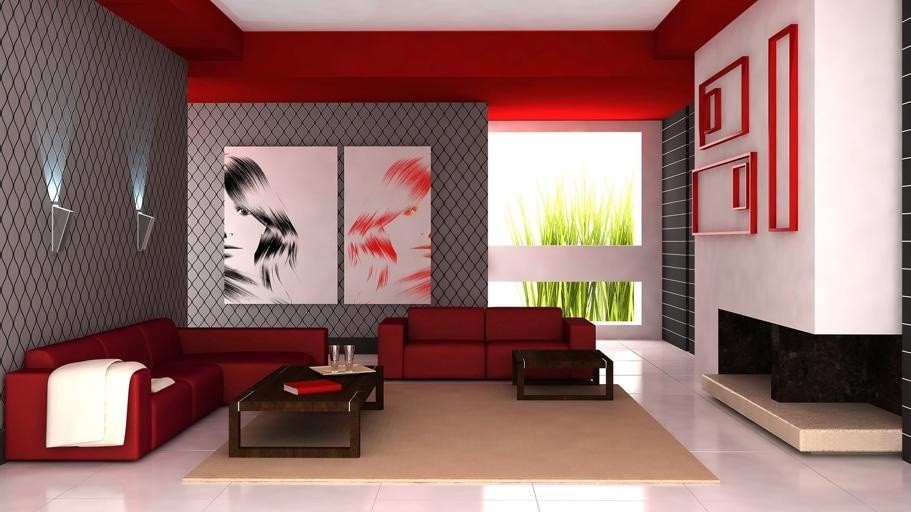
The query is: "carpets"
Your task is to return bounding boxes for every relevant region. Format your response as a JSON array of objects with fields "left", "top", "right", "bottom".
[{"left": 181, "top": 367, "right": 720, "bottom": 485}]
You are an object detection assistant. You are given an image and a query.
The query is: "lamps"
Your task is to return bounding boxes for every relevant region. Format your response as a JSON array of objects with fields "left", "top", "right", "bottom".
[
  {"left": 52, "top": 204, "right": 76, "bottom": 253},
  {"left": 136, "top": 211, "right": 155, "bottom": 257}
]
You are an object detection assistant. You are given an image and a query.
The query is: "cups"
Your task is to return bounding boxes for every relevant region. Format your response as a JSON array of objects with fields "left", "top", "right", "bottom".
[
  {"left": 331, "top": 360, "right": 338, "bottom": 373},
  {"left": 344, "top": 345, "right": 355, "bottom": 364},
  {"left": 345, "top": 361, "right": 353, "bottom": 372},
  {"left": 329, "top": 345, "right": 340, "bottom": 361}
]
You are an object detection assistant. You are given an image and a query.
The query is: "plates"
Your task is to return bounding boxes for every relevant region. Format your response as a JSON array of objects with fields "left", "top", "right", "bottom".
[{"left": 309, "top": 364, "right": 376, "bottom": 375}]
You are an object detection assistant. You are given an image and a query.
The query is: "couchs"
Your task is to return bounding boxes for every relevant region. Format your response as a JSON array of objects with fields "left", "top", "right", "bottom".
[
  {"left": 378, "top": 306, "right": 597, "bottom": 380},
  {"left": 179, "top": 327, "right": 329, "bottom": 405},
  {"left": 4, "top": 318, "right": 225, "bottom": 462}
]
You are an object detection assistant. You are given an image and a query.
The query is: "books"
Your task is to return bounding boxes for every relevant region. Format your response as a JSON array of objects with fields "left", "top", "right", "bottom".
[{"left": 282, "top": 379, "right": 342, "bottom": 397}]
